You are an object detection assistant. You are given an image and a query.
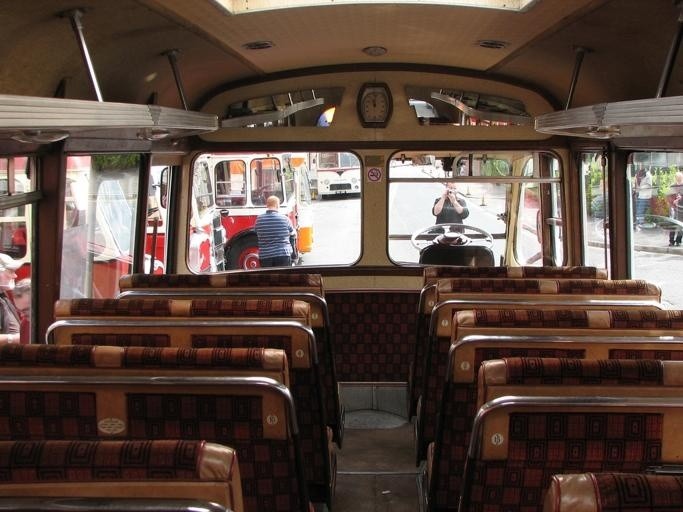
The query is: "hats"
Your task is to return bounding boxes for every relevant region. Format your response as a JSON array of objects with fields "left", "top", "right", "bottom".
[{"left": 0, "top": 253, "right": 25, "bottom": 271}]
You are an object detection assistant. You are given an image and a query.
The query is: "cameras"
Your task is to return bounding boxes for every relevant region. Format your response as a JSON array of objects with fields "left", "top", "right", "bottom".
[{"left": 447, "top": 190, "right": 454, "bottom": 195}]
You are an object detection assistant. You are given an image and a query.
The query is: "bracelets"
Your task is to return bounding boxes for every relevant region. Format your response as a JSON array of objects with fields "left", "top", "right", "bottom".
[{"left": 8, "top": 333, "right": 13, "bottom": 344}]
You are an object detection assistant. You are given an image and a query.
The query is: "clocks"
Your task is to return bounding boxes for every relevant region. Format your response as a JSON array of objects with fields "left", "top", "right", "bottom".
[{"left": 353, "top": 82, "right": 394, "bottom": 128}]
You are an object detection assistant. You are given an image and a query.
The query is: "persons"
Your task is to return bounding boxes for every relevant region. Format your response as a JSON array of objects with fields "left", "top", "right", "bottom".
[
  {"left": 11, "top": 224, "right": 26, "bottom": 258},
  {"left": 12, "top": 278, "right": 85, "bottom": 345},
  {"left": 631, "top": 163, "right": 653, "bottom": 232},
  {"left": 254, "top": 195, "right": 297, "bottom": 267},
  {"left": 667, "top": 172, "right": 683, "bottom": 247},
  {"left": 60, "top": 204, "right": 106, "bottom": 299},
  {"left": 0, "top": 253, "right": 22, "bottom": 347},
  {"left": 432, "top": 182, "right": 469, "bottom": 234}
]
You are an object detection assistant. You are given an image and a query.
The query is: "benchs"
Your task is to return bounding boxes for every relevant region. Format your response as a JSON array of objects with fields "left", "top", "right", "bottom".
[
  {"left": 408, "top": 266, "right": 683, "bottom": 511},
  {"left": 0, "top": 275, "right": 334, "bottom": 511}
]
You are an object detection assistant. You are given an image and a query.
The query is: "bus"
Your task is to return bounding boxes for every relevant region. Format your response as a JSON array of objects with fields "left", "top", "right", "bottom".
[{"left": 316, "top": 150, "right": 361, "bottom": 200}]
